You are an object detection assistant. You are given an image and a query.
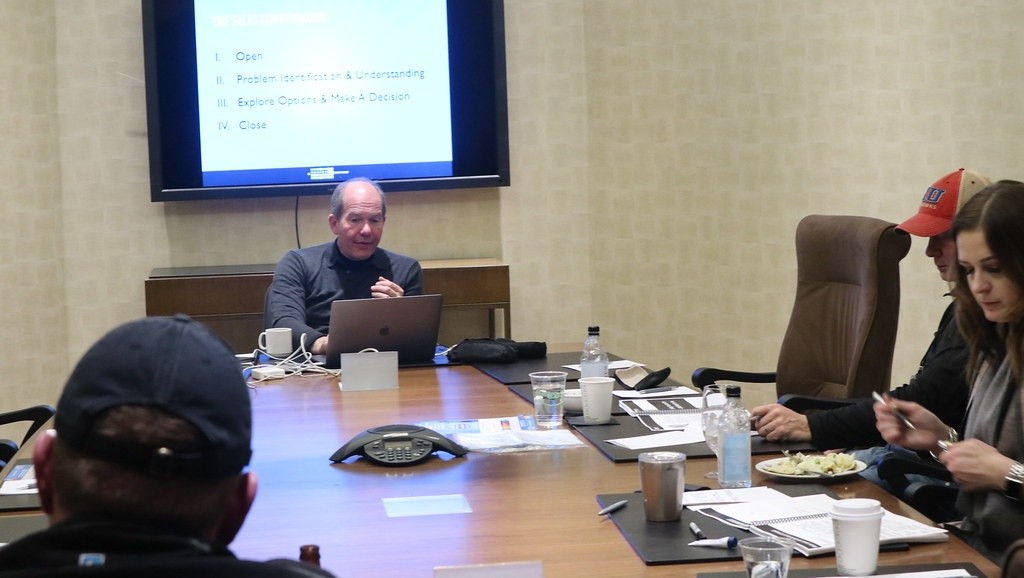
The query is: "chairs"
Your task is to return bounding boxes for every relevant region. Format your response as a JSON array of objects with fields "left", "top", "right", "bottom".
[{"left": 692, "top": 216, "right": 915, "bottom": 412}]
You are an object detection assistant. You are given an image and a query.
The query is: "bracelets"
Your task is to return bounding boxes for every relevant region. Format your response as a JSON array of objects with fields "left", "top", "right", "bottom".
[{"left": 930, "top": 427, "right": 958, "bottom": 463}]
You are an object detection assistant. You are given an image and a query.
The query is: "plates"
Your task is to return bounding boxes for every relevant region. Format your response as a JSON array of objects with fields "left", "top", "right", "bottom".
[{"left": 755, "top": 456, "right": 867, "bottom": 481}]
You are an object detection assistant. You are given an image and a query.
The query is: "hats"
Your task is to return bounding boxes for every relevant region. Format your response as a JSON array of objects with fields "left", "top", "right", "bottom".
[
  {"left": 895, "top": 168, "right": 992, "bottom": 237},
  {"left": 55, "top": 313, "right": 252, "bottom": 474}
]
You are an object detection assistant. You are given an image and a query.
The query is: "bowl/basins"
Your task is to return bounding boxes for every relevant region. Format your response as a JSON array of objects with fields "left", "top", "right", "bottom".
[{"left": 563, "top": 389, "right": 583, "bottom": 414}]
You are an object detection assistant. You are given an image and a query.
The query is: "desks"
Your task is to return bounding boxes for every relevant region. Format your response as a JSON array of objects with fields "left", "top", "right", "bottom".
[
  {"left": 144, "top": 257, "right": 513, "bottom": 339},
  {"left": 0, "top": 354, "right": 1001, "bottom": 578}
]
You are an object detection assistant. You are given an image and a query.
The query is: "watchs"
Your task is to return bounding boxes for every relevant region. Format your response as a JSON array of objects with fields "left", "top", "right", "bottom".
[{"left": 1004, "top": 462, "right": 1024, "bottom": 499}]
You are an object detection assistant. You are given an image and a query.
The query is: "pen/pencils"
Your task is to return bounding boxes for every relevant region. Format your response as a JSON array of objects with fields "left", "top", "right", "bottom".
[
  {"left": 598, "top": 500, "right": 629, "bottom": 516},
  {"left": 872, "top": 391, "right": 916, "bottom": 431},
  {"left": 689, "top": 522, "right": 707, "bottom": 540}
]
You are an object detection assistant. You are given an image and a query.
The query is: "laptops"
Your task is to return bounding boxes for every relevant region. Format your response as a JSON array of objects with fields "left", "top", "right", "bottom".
[{"left": 310, "top": 293, "right": 445, "bottom": 369}]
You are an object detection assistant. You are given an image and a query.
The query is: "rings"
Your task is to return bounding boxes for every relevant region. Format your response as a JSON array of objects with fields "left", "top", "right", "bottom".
[{"left": 764, "top": 426, "right": 769, "bottom": 433}]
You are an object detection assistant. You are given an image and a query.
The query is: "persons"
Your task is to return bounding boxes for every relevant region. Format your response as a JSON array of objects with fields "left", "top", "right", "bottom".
[
  {"left": 749, "top": 167, "right": 1024, "bottom": 567},
  {"left": 0, "top": 310, "right": 334, "bottom": 577},
  {"left": 265, "top": 177, "right": 422, "bottom": 356}
]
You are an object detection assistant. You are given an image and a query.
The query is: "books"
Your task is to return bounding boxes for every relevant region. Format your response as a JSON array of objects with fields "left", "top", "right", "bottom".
[
  {"left": 700, "top": 493, "right": 950, "bottom": 557},
  {"left": 619, "top": 397, "right": 750, "bottom": 431},
  {"left": 0, "top": 466, "right": 42, "bottom": 509}
]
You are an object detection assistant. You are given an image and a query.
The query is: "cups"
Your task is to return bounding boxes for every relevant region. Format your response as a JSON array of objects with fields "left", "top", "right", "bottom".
[
  {"left": 528, "top": 371, "right": 569, "bottom": 427},
  {"left": 578, "top": 376, "right": 615, "bottom": 425},
  {"left": 828, "top": 498, "right": 886, "bottom": 577},
  {"left": 737, "top": 536, "right": 794, "bottom": 578},
  {"left": 258, "top": 328, "right": 293, "bottom": 358},
  {"left": 637, "top": 451, "right": 686, "bottom": 522}
]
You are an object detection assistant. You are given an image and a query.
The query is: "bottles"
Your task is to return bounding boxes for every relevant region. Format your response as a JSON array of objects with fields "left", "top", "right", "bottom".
[
  {"left": 581, "top": 326, "right": 610, "bottom": 378},
  {"left": 716, "top": 385, "right": 752, "bottom": 488}
]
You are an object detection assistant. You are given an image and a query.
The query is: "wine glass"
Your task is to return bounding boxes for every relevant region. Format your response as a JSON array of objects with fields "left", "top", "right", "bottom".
[{"left": 701, "top": 384, "right": 743, "bottom": 479}]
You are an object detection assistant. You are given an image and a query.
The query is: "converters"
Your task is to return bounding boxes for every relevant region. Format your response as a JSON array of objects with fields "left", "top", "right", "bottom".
[{"left": 251, "top": 366, "right": 285, "bottom": 380}]
